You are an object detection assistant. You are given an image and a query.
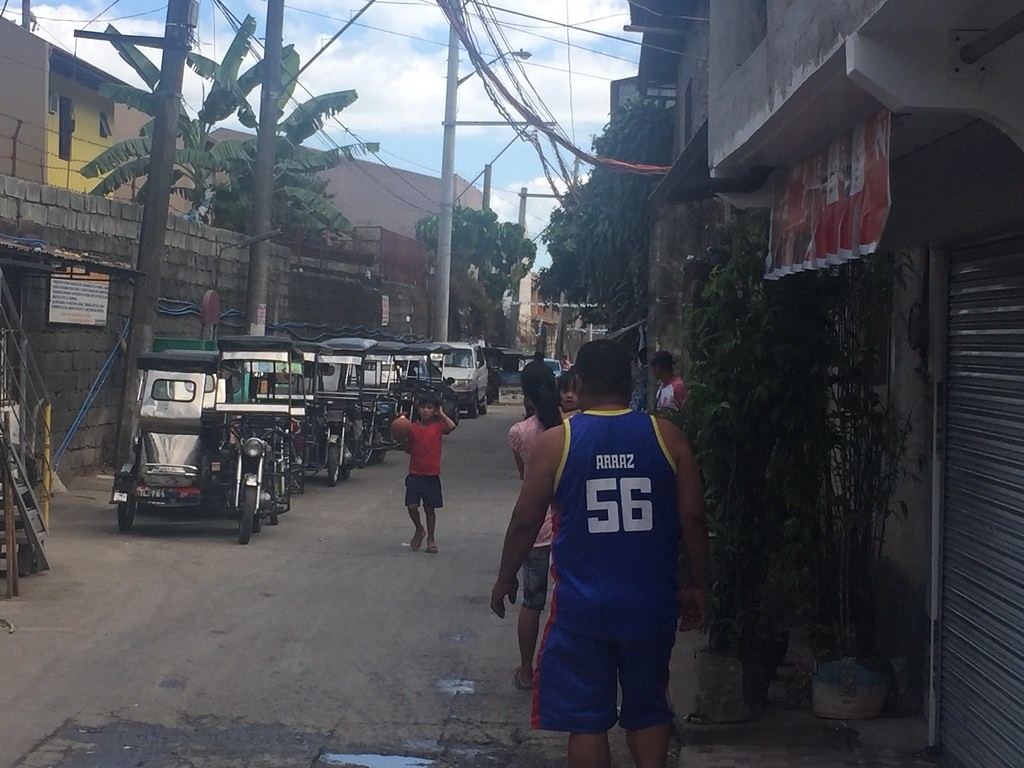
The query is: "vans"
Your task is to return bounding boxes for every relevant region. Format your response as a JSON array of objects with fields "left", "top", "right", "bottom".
[{"left": 423, "top": 342, "right": 489, "bottom": 418}]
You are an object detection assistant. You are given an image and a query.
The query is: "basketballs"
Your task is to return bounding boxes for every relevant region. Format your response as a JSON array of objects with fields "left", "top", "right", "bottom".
[{"left": 390, "top": 417, "right": 413, "bottom": 441}]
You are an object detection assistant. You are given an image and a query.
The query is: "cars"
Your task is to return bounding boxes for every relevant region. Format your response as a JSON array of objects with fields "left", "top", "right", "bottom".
[
  {"left": 408, "top": 364, "right": 460, "bottom": 425},
  {"left": 525, "top": 359, "right": 569, "bottom": 379}
]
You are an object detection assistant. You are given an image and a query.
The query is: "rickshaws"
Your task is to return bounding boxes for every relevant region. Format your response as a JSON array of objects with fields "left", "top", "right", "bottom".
[
  {"left": 393, "top": 343, "right": 454, "bottom": 423},
  {"left": 342, "top": 341, "right": 409, "bottom": 463},
  {"left": 108, "top": 335, "right": 307, "bottom": 544},
  {"left": 257, "top": 338, "right": 377, "bottom": 486}
]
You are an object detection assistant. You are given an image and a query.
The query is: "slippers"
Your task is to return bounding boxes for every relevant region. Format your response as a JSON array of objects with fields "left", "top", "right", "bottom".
[
  {"left": 425, "top": 539, "right": 439, "bottom": 553},
  {"left": 410, "top": 529, "right": 426, "bottom": 551},
  {"left": 514, "top": 665, "right": 535, "bottom": 689}
]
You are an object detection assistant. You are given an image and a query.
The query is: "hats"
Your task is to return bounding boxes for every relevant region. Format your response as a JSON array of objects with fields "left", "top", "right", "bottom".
[
  {"left": 643, "top": 351, "right": 674, "bottom": 367},
  {"left": 569, "top": 339, "right": 632, "bottom": 392}
]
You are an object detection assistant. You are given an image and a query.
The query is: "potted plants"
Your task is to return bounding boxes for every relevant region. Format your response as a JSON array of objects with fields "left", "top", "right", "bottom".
[{"left": 679, "top": 241, "right": 889, "bottom": 719}]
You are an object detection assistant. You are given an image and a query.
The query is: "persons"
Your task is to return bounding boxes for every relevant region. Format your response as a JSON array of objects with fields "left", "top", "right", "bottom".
[
  {"left": 490, "top": 340, "right": 709, "bottom": 768},
  {"left": 509, "top": 361, "right": 569, "bottom": 691},
  {"left": 562, "top": 355, "right": 569, "bottom": 369},
  {"left": 556, "top": 371, "right": 580, "bottom": 412},
  {"left": 401, "top": 392, "right": 458, "bottom": 554},
  {"left": 650, "top": 350, "right": 686, "bottom": 412}
]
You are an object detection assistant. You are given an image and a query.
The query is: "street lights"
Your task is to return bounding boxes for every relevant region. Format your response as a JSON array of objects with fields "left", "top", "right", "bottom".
[{"left": 436, "top": 48, "right": 532, "bottom": 343}]
